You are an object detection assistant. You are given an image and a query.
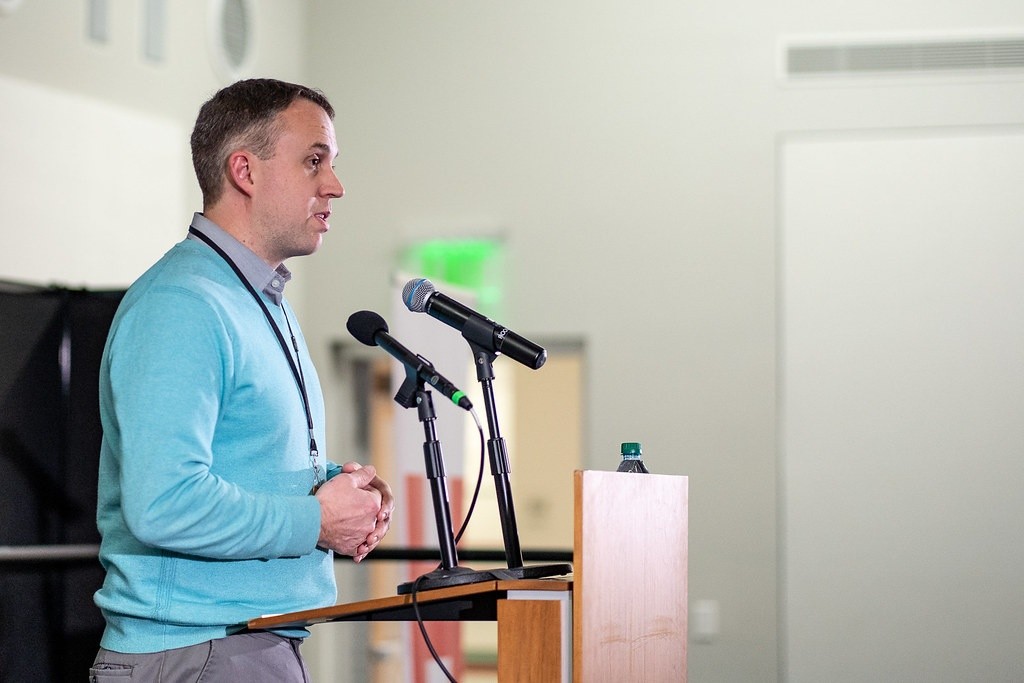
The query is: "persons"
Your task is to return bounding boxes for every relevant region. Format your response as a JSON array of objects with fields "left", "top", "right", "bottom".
[{"left": 89, "top": 79, "right": 395, "bottom": 682}]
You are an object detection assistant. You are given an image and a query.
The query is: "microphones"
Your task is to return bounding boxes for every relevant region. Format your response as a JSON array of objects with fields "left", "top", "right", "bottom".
[
  {"left": 403, "top": 278, "right": 548, "bottom": 370},
  {"left": 347, "top": 310, "right": 473, "bottom": 410}
]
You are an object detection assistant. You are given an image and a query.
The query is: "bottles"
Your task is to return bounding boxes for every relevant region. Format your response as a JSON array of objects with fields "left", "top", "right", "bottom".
[{"left": 615, "top": 442, "right": 650, "bottom": 474}]
